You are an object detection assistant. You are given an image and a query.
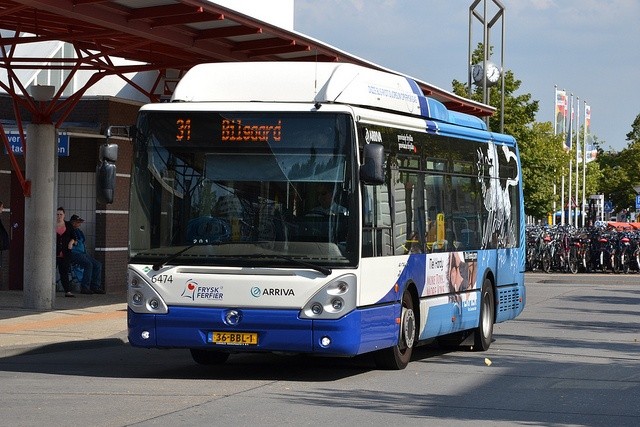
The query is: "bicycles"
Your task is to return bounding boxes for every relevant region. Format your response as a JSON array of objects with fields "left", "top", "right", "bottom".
[{"left": 524, "top": 224, "right": 640, "bottom": 273}]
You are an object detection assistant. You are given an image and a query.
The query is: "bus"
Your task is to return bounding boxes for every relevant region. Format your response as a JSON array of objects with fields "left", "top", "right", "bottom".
[{"left": 96, "top": 61, "right": 526, "bottom": 370}]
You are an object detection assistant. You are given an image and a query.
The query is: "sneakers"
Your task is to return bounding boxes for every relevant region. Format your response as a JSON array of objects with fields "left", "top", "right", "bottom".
[
  {"left": 93, "top": 288, "right": 105, "bottom": 293},
  {"left": 80, "top": 289, "right": 93, "bottom": 293}
]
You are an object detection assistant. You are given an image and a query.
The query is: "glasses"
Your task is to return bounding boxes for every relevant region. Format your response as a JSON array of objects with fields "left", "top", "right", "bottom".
[
  {"left": 57, "top": 214, "right": 64, "bottom": 215},
  {"left": 317, "top": 191, "right": 330, "bottom": 196}
]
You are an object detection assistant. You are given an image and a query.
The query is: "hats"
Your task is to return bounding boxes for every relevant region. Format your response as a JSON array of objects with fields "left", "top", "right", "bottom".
[{"left": 70, "top": 214, "right": 84, "bottom": 223}]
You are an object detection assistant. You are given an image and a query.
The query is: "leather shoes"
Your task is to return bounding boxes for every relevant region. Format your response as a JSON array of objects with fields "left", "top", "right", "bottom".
[{"left": 65, "top": 294, "right": 77, "bottom": 297}]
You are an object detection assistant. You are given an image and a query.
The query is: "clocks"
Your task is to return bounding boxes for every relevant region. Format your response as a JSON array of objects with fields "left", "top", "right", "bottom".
[
  {"left": 473, "top": 65, "right": 483, "bottom": 82},
  {"left": 486, "top": 66, "right": 500, "bottom": 82}
]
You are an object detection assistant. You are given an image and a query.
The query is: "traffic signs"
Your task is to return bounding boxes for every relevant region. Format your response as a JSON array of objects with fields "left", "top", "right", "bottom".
[{"left": 2, "top": 132, "right": 71, "bottom": 157}]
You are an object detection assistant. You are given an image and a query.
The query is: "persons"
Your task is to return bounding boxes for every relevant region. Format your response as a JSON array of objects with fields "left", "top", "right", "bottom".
[
  {"left": 68, "top": 215, "right": 104, "bottom": 294},
  {"left": 308, "top": 184, "right": 354, "bottom": 259},
  {"left": 54, "top": 206, "right": 74, "bottom": 297}
]
